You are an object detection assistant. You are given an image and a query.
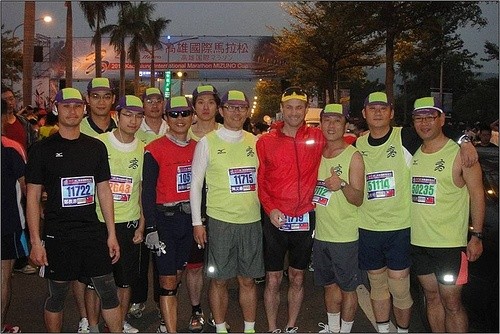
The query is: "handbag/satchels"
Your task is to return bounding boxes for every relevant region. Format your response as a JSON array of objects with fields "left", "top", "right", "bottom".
[{"left": 156, "top": 201, "right": 192, "bottom": 216}]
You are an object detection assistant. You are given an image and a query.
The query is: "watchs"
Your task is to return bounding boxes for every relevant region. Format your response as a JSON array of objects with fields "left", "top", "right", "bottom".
[
  {"left": 472, "top": 232, "right": 485, "bottom": 239},
  {"left": 340, "top": 180, "right": 346, "bottom": 189},
  {"left": 462, "top": 136, "right": 470, "bottom": 142}
]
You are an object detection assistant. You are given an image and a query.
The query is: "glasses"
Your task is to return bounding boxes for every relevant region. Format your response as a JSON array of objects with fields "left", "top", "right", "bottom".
[
  {"left": 283, "top": 88, "right": 308, "bottom": 97},
  {"left": 122, "top": 113, "right": 145, "bottom": 120},
  {"left": 167, "top": 109, "right": 192, "bottom": 118},
  {"left": 143, "top": 100, "right": 163, "bottom": 105},
  {"left": 224, "top": 105, "right": 248, "bottom": 112},
  {"left": 89, "top": 94, "right": 113, "bottom": 100},
  {"left": 413, "top": 114, "right": 439, "bottom": 123},
  {"left": 368, "top": 107, "right": 391, "bottom": 113}
]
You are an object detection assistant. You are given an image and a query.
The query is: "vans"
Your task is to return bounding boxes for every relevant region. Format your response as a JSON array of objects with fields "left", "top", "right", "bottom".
[{"left": 275, "top": 108, "right": 324, "bottom": 128}]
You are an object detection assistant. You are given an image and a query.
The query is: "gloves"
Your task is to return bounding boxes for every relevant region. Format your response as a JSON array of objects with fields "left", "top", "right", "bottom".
[{"left": 144, "top": 227, "right": 166, "bottom": 257}]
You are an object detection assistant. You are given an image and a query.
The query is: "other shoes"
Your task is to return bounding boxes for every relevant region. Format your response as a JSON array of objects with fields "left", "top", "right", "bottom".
[{"left": 254, "top": 276, "right": 265, "bottom": 285}]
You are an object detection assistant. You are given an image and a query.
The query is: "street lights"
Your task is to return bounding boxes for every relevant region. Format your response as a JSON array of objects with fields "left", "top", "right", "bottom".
[
  {"left": 11, "top": 17, "right": 53, "bottom": 89},
  {"left": 155, "top": 35, "right": 198, "bottom": 112}
]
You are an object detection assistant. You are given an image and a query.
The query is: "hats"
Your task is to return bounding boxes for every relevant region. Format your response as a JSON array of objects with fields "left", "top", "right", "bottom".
[
  {"left": 53, "top": 88, "right": 85, "bottom": 104},
  {"left": 412, "top": 97, "right": 443, "bottom": 117},
  {"left": 143, "top": 88, "right": 164, "bottom": 99},
  {"left": 364, "top": 92, "right": 394, "bottom": 109},
  {"left": 192, "top": 85, "right": 250, "bottom": 105},
  {"left": 87, "top": 77, "right": 115, "bottom": 92},
  {"left": 164, "top": 96, "right": 193, "bottom": 111},
  {"left": 279, "top": 86, "right": 309, "bottom": 104},
  {"left": 320, "top": 104, "right": 349, "bottom": 120},
  {"left": 115, "top": 95, "right": 147, "bottom": 111}
]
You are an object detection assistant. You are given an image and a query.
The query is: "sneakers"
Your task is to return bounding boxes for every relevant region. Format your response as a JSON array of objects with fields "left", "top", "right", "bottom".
[
  {"left": 14, "top": 264, "right": 38, "bottom": 273},
  {"left": 156, "top": 318, "right": 167, "bottom": 334},
  {"left": 127, "top": 302, "right": 145, "bottom": 320},
  {"left": 105, "top": 320, "right": 140, "bottom": 334},
  {"left": 0, "top": 322, "right": 22, "bottom": 334},
  {"left": 187, "top": 310, "right": 231, "bottom": 334},
  {"left": 78, "top": 318, "right": 91, "bottom": 334}
]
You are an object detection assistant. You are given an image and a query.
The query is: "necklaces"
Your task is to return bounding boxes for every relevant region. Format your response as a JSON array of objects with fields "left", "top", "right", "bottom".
[{"left": 118, "top": 128, "right": 126, "bottom": 144}]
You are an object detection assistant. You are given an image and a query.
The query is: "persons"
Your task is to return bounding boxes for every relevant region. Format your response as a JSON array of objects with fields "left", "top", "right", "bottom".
[{"left": 0, "top": 74, "right": 500, "bottom": 333}]
